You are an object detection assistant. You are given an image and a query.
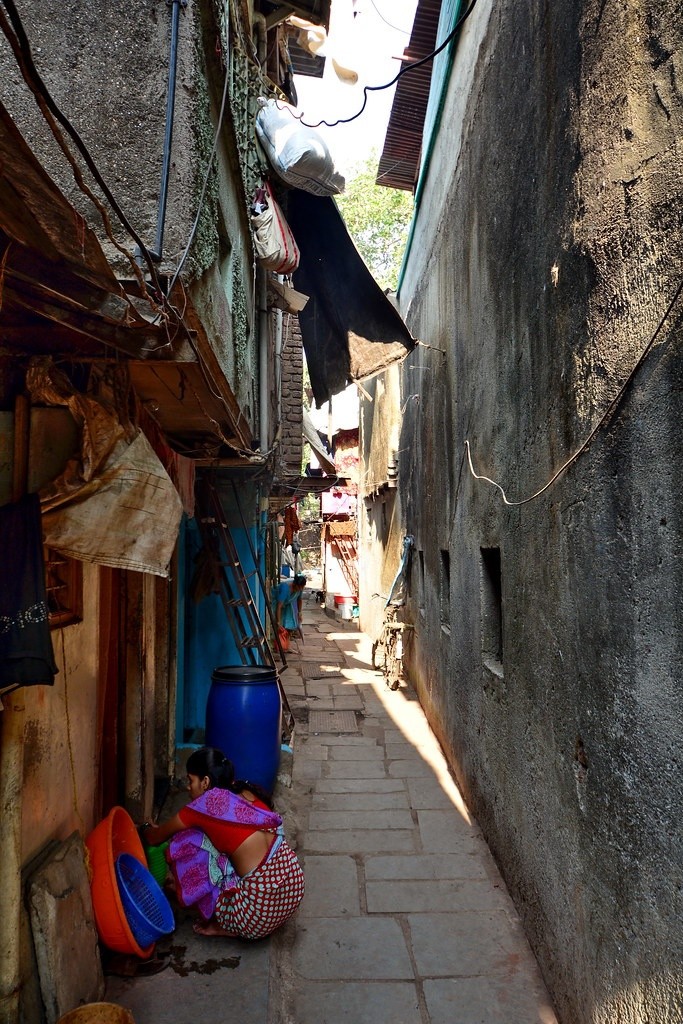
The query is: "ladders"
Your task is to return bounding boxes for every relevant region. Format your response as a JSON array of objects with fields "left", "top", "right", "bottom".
[
  {"left": 192, "top": 487, "right": 295, "bottom": 739},
  {"left": 335, "top": 536, "right": 359, "bottom": 590}
]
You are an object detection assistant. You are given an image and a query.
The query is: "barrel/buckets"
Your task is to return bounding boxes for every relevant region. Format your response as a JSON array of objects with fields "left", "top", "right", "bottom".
[
  {"left": 204, "top": 665, "right": 281, "bottom": 795},
  {"left": 338, "top": 604, "right": 354, "bottom": 619}
]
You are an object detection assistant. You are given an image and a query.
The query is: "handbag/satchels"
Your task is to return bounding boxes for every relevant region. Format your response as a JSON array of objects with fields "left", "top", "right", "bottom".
[{"left": 274, "top": 625, "right": 289, "bottom": 654}]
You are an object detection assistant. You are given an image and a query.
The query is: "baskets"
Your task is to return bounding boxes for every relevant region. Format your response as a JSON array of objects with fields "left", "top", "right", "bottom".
[
  {"left": 114, "top": 853, "right": 175, "bottom": 948},
  {"left": 146, "top": 842, "right": 168, "bottom": 889}
]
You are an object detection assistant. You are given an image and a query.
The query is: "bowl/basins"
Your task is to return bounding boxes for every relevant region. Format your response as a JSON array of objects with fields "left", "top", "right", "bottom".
[
  {"left": 85, "top": 807, "right": 155, "bottom": 958},
  {"left": 334, "top": 596, "right": 356, "bottom": 604},
  {"left": 56, "top": 1001, "right": 135, "bottom": 1024}
]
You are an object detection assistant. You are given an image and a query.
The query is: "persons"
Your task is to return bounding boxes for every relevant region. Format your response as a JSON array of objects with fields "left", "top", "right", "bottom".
[
  {"left": 271, "top": 574, "right": 306, "bottom": 653},
  {"left": 143, "top": 748, "right": 305, "bottom": 941}
]
